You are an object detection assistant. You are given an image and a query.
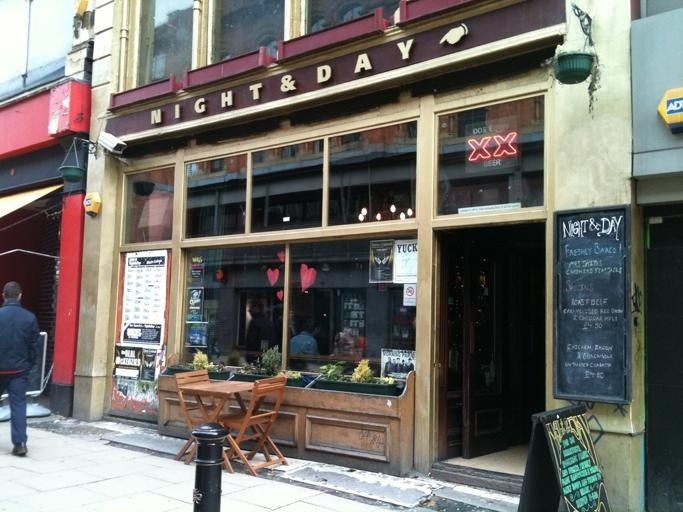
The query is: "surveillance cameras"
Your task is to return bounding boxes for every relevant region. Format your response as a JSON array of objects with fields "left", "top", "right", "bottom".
[{"left": 98, "top": 131, "right": 126, "bottom": 155}]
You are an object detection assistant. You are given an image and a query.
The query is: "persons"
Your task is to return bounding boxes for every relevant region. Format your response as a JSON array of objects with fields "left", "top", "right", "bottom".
[
  {"left": 290, "top": 321, "right": 321, "bottom": 369},
  {"left": 245, "top": 298, "right": 278, "bottom": 365},
  {"left": 0, "top": 282, "right": 39, "bottom": 457},
  {"left": 384, "top": 357, "right": 414, "bottom": 373}
]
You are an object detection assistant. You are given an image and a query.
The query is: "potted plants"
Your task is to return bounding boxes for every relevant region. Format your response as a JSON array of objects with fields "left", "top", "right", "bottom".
[{"left": 165, "top": 344, "right": 399, "bottom": 395}]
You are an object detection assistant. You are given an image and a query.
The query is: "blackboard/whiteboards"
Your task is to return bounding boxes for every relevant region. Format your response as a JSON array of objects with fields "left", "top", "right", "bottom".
[
  {"left": 340, "top": 292, "right": 365, "bottom": 338},
  {"left": 1, "top": 331, "right": 48, "bottom": 398},
  {"left": 552, "top": 204, "right": 633, "bottom": 406},
  {"left": 540, "top": 404, "right": 611, "bottom": 512}
]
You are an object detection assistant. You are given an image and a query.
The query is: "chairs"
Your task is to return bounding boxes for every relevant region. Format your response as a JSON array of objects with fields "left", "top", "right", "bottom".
[{"left": 174, "top": 369, "right": 288, "bottom": 477}]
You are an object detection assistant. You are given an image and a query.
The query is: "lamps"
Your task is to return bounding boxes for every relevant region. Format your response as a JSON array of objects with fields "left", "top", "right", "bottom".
[{"left": 541, "top": 4, "right": 601, "bottom": 120}]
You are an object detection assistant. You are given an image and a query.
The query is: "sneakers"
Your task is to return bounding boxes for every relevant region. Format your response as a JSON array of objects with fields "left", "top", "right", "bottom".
[{"left": 11, "top": 441, "right": 28, "bottom": 456}]
